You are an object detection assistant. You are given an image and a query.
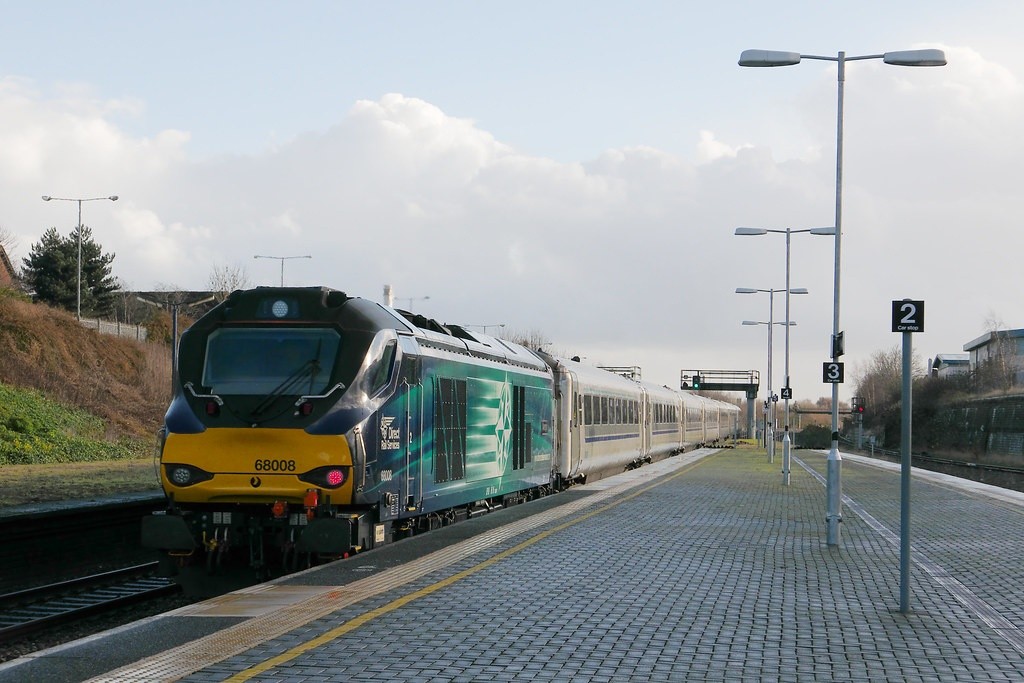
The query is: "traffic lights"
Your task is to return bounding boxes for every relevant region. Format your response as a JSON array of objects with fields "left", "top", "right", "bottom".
[
  {"left": 693, "top": 376, "right": 701, "bottom": 390},
  {"left": 858, "top": 406, "right": 863, "bottom": 412}
]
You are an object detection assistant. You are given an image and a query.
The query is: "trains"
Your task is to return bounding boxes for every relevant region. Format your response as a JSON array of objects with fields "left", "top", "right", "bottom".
[{"left": 155, "top": 284, "right": 742, "bottom": 582}]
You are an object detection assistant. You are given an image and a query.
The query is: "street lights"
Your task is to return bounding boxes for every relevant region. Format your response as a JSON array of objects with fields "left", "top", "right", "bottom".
[
  {"left": 734, "top": 287, "right": 808, "bottom": 463},
  {"left": 528, "top": 343, "right": 553, "bottom": 348},
  {"left": 742, "top": 321, "right": 798, "bottom": 462},
  {"left": 468, "top": 325, "right": 505, "bottom": 334},
  {"left": 736, "top": 227, "right": 842, "bottom": 482},
  {"left": 254, "top": 254, "right": 312, "bottom": 286},
  {"left": 43, "top": 196, "right": 119, "bottom": 320},
  {"left": 738, "top": 49, "right": 951, "bottom": 543},
  {"left": 393, "top": 297, "right": 429, "bottom": 310}
]
